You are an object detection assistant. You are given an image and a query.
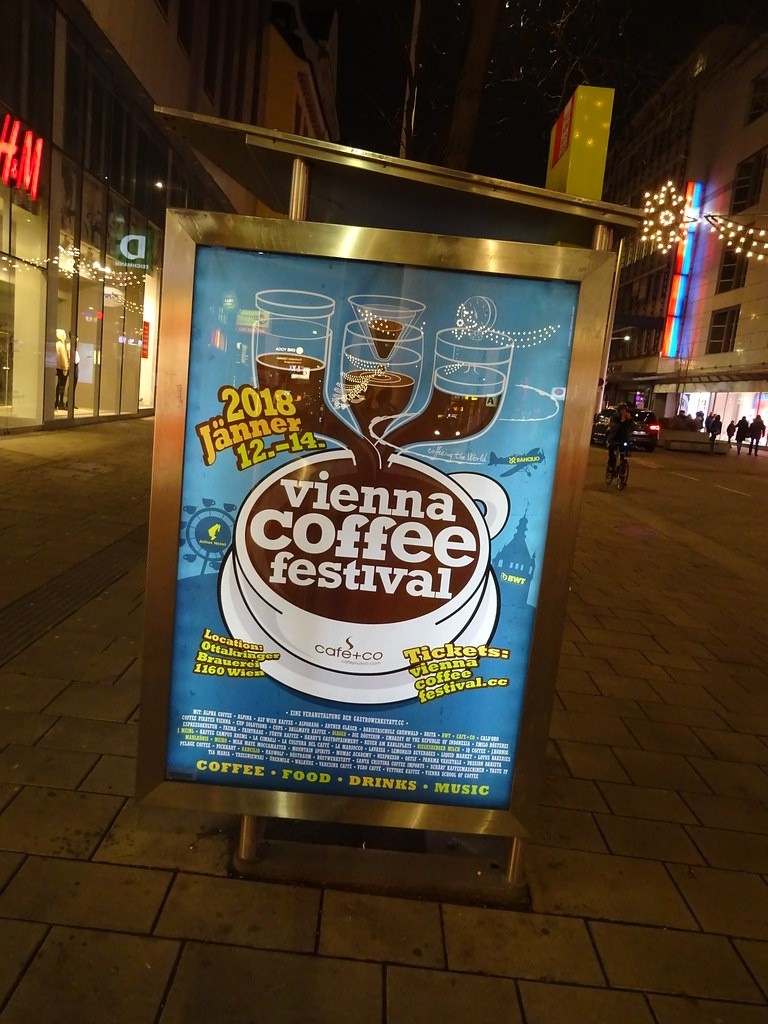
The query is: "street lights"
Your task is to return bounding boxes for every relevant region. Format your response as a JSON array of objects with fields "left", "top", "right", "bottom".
[{"left": 598, "top": 336, "right": 629, "bottom": 411}]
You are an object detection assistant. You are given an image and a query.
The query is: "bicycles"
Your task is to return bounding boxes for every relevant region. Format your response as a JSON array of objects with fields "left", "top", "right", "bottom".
[{"left": 606, "top": 439, "right": 635, "bottom": 489}]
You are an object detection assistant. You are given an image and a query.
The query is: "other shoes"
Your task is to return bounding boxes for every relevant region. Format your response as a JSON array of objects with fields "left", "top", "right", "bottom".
[{"left": 607, "top": 465, "right": 615, "bottom": 473}]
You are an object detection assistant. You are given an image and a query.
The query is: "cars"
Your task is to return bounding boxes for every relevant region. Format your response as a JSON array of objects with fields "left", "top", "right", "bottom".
[{"left": 591, "top": 402, "right": 659, "bottom": 452}]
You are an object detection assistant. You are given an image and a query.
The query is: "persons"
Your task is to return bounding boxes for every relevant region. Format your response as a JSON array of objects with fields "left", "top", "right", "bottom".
[
  {"left": 56, "top": 329, "right": 80, "bottom": 410},
  {"left": 607, "top": 402, "right": 636, "bottom": 473},
  {"left": 677, "top": 410, "right": 704, "bottom": 432},
  {"left": 726, "top": 421, "right": 735, "bottom": 448},
  {"left": 706, "top": 412, "right": 722, "bottom": 452},
  {"left": 748, "top": 415, "right": 766, "bottom": 456},
  {"left": 735, "top": 416, "right": 749, "bottom": 454}
]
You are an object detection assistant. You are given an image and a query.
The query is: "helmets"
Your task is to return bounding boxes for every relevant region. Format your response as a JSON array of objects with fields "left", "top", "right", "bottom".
[{"left": 617, "top": 402, "right": 628, "bottom": 410}]
